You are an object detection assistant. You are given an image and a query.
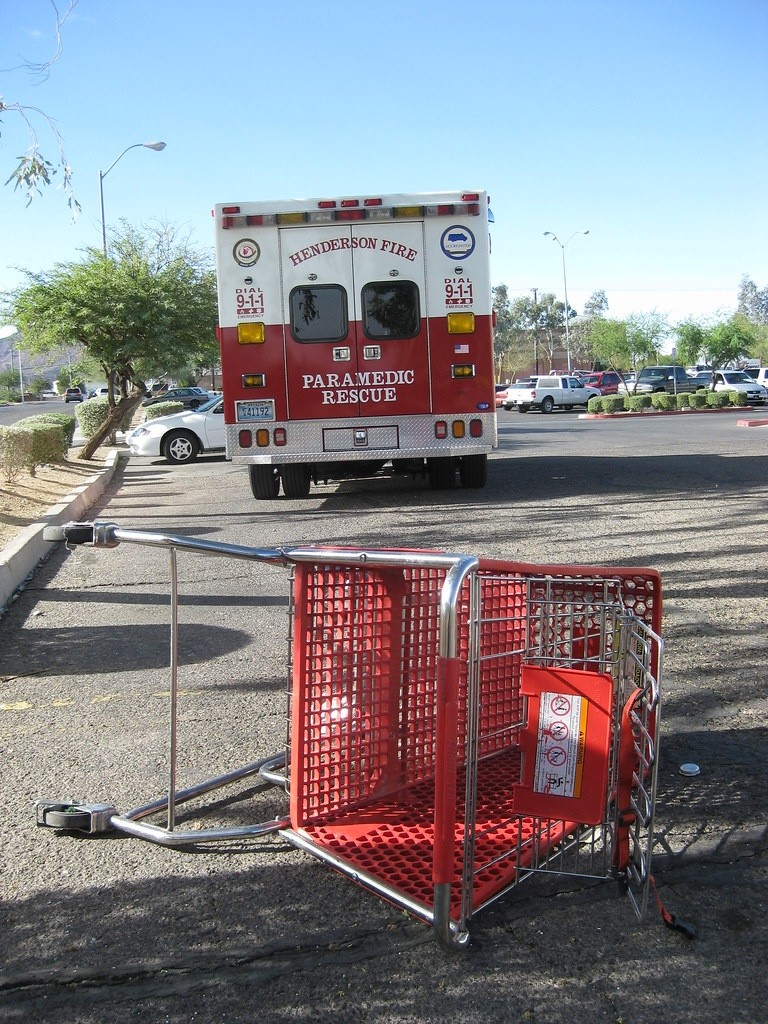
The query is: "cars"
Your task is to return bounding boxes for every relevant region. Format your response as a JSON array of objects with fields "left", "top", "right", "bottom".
[
  {"left": 140, "top": 384, "right": 223, "bottom": 410},
  {"left": 64, "top": 388, "right": 83, "bottom": 403},
  {"left": 496, "top": 364, "right": 768, "bottom": 411},
  {"left": 126, "top": 394, "right": 225, "bottom": 464},
  {"left": 87, "top": 387, "right": 108, "bottom": 399}
]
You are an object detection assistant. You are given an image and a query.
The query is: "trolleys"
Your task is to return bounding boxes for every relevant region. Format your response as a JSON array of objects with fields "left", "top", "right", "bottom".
[{"left": 32, "top": 520, "right": 699, "bottom": 953}]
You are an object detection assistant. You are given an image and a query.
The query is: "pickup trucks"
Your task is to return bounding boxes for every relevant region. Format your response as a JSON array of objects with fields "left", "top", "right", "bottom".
[{"left": 503, "top": 373, "right": 601, "bottom": 413}]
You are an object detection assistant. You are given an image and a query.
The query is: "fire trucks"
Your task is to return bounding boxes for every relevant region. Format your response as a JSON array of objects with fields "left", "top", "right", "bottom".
[{"left": 210, "top": 189, "right": 500, "bottom": 500}]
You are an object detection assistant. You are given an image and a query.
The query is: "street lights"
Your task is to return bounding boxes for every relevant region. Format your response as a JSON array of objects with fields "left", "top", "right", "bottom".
[
  {"left": 530, "top": 287, "right": 542, "bottom": 375},
  {"left": 541, "top": 229, "right": 588, "bottom": 370},
  {"left": 97, "top": 140, "right": 166, "bottom": 278}
]
